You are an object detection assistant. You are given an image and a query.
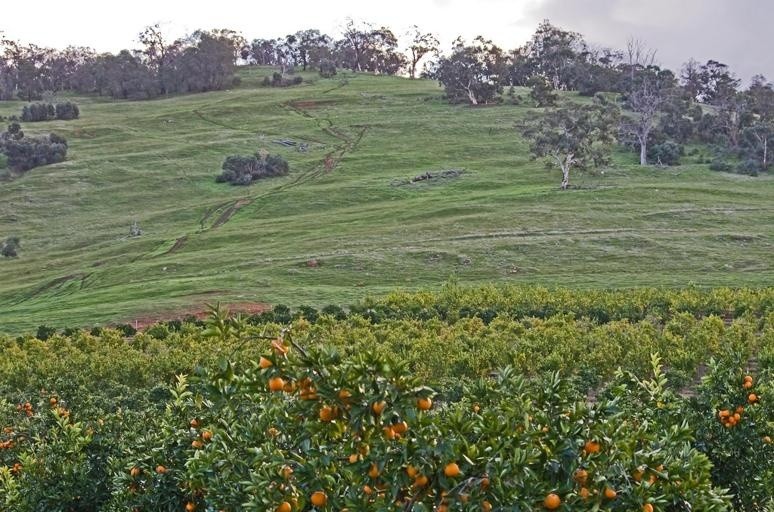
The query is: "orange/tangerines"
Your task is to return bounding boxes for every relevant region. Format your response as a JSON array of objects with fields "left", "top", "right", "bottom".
[{"left": 0, "top": 337, "right": 771, "bottom": 512}]
